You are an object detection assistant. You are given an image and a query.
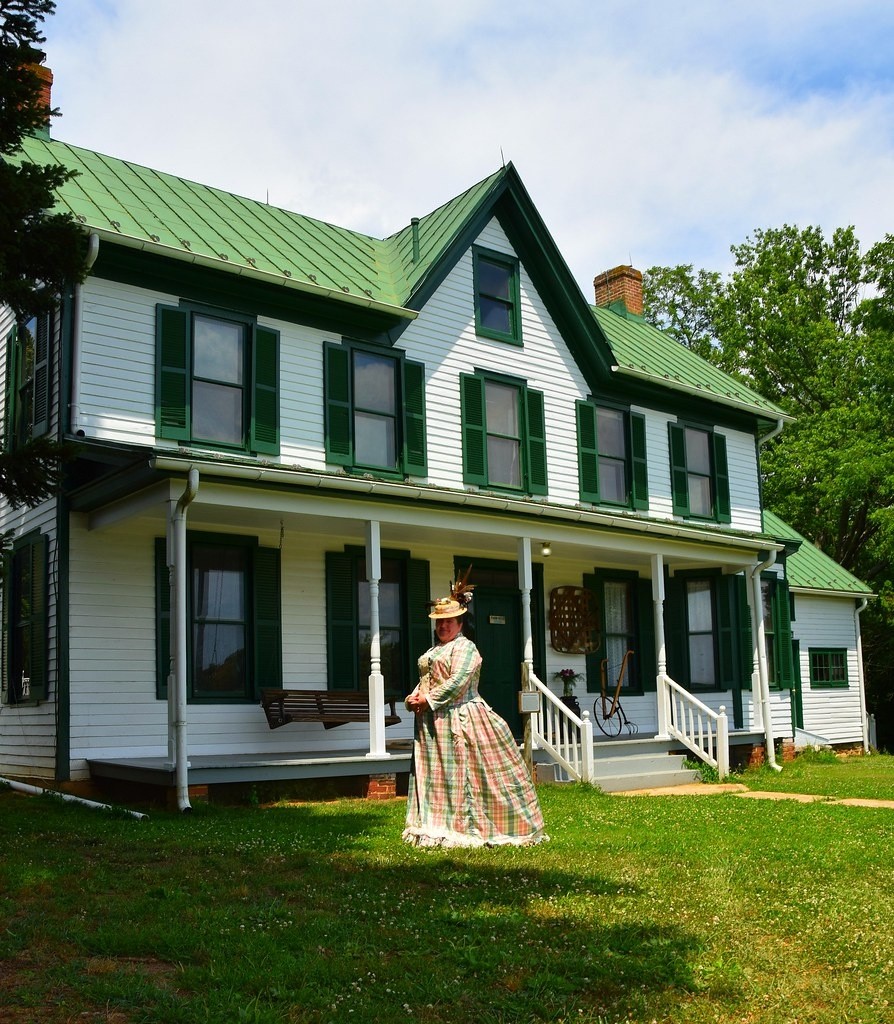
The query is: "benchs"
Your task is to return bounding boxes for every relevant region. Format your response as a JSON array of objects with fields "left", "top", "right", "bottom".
[{"left": 263, "top": 688, "right": 402, "bottom": 732}]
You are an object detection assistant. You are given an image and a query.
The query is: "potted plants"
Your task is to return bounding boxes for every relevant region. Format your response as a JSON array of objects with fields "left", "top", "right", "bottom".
[{"left": 554, "top": 668, "right": 584, "bottom": 696}]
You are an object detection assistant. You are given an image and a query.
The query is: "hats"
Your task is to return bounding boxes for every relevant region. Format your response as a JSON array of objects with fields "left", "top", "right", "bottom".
[{"left": 427, "top": 564, "right": 474, "bottom": 618}]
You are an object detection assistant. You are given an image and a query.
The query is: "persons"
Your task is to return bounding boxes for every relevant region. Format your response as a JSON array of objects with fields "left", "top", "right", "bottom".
[{"left": 401, "top": 598, "right": 551, "bottom": 851}]
